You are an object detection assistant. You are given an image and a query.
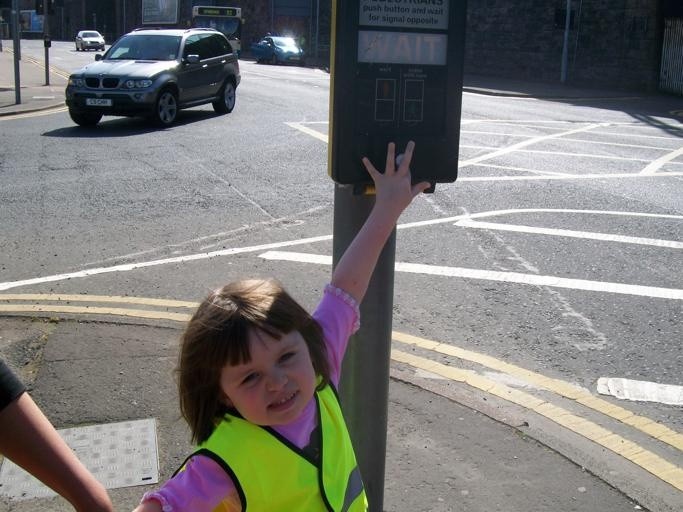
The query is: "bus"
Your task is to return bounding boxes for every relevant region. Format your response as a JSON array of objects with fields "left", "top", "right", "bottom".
[{"left": 181, "top": 4, "right": 246, "bottom": 58}]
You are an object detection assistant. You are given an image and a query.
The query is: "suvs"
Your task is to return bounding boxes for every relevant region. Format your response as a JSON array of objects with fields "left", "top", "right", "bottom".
[{"left": 66, "top": 27, "right": 240, "bottom": 125}]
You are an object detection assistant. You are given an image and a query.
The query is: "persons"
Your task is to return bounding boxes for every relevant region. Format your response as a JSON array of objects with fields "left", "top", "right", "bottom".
[
  {"left": 132, "top": 140, "right": 431, "bottom": 511},
  {"left": 0, "top": 359, "right": 114, "bottom": 512}
]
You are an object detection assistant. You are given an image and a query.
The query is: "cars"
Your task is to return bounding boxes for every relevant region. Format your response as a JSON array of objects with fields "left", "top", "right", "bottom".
[
  {"left": 75, "top": 28, "right": 104, "bottom": 51},
  {"left": 252, "top": 35, "right": 304, "bottom": 65}
]
